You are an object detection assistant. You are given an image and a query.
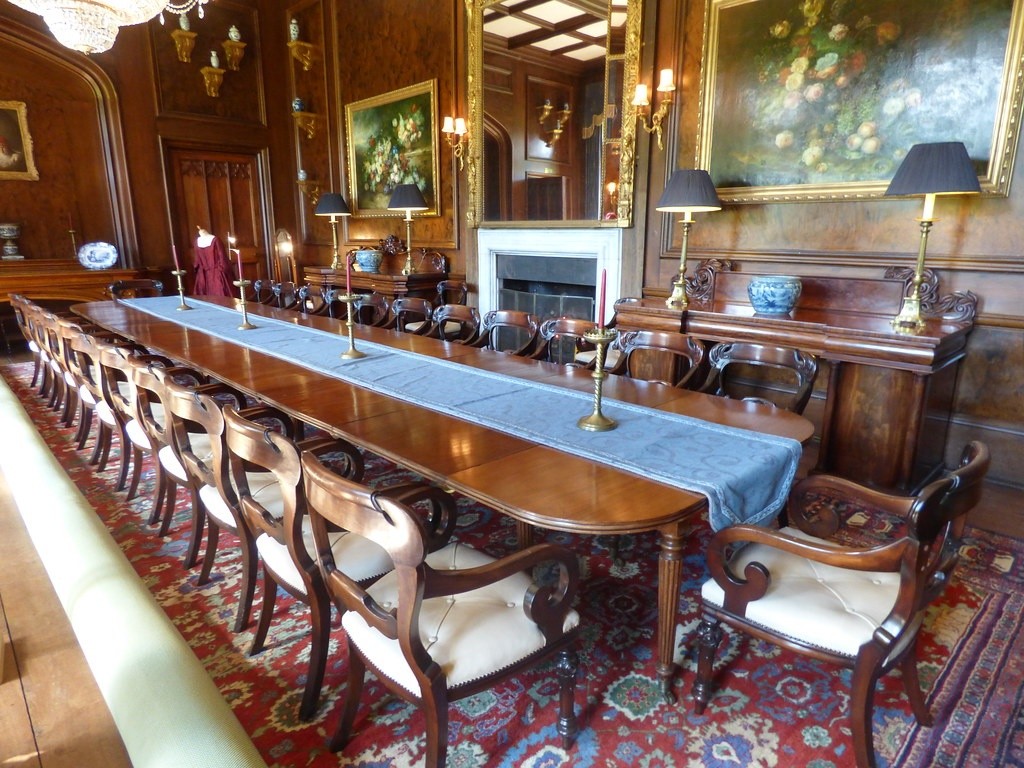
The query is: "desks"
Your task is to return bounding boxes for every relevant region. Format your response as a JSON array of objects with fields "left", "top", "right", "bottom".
[
  {"left": 0, "top": 258, "right": 139, "bottom": 359},
  {"left": 66, "top": 291, "right": 816, "bottom": 696},
  {"left": 304, "top": 234, "right": 446, "bottom": 333},
  {"left": 614, "top": 258, "right": 974, "bottom": 499}
]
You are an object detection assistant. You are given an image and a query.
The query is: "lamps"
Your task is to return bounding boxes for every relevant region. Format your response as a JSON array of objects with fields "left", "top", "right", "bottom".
[
  {"left": 441, "top": 114, "right": 468, "bottom": 172},
  {"left": 884, "top": 140, "right": 984, "bottom": 329},
  {"left": 6, "top": 0, "right": 208, "bottom": 54},
  {"left": 314, "top": 193, "right": 351, "bottom": 269},
  {"left": 654, "top": 169, "right": 724, "bottom": 306},
  {"left": 629, "top": 68, "right": 683, "bottom": 149},
  {"left": 387, "top": 185, "right": 430, "bottom": 271}
]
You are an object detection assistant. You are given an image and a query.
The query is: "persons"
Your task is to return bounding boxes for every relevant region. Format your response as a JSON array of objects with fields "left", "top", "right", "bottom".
[{"left": 193, "top": 225, "right": 240, "bottom": 298}]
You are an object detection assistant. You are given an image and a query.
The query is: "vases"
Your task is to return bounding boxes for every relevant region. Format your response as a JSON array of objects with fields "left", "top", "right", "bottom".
[
  {"left": 563, "top": 103, "right": 570, "bottom": 110},
  {"left": 544, "top": 99, "right": 551, "bottom": 106},
  {"left": 748, "top": 276, "right": 804, "bottom": 317},
  {"left": 555, "top": 120, "right": 563, "bottom": 129},
  {"left": 355, "top": 250, "right": 384, "bottom": 271}
]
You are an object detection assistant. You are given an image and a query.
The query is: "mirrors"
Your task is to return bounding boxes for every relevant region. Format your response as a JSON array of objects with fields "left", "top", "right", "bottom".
[
  {"left": 274, "top": 227, "right": 298, "bottom": 284},
  {"left": 462, "top": 0, "right": 643, "bottom": 230}
]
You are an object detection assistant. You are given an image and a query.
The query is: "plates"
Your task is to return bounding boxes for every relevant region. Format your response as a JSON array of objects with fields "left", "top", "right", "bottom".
[{"left": 78, "top": 242, "right": 118, "bottom": 269}]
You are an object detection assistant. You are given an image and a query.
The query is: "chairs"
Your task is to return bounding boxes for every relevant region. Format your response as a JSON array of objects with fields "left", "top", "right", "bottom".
[{"left": 6, "top": 270, "right": 992, "bottom": 768}]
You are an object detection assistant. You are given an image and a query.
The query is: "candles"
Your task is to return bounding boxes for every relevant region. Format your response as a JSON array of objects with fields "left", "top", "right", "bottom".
[
  {"left": 235, "top": 249, "right": 244, "bottom": 280},
  {"left": 598, "top": 267, "right": 609, "bottom": 328},
  {"left": 346, "top": 256, "right": 352, "bottom": 294},
  {"left": 67, "top": 211, "right": 74, "bottom": 230},
  {"left": 172, "top": 244, "right": 181, "bottom": 268}
]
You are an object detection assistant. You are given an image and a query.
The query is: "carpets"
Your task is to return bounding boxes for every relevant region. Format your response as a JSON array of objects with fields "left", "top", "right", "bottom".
[{"left": 0, "top": 358, "right": 1024, "bottom": 768}]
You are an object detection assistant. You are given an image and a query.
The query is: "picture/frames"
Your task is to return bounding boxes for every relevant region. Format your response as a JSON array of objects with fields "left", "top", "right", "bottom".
[
  {"left": 0, "top": 100, "right": 40, "bottom": 182},
  {"left": 343, "top": 76, "right": 444, "bottom": 219},
  {"left": 693, "top": 0, "right": 1024, "bottom": 206}
]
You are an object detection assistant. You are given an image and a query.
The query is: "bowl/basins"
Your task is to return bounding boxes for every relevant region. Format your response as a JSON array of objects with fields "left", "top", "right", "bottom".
[
  {"left": 747, "top": 275, "right": 802, "bottom": 316},
  {"left": 355, "top": 249, "right": 382, "bottom": 272}
]
